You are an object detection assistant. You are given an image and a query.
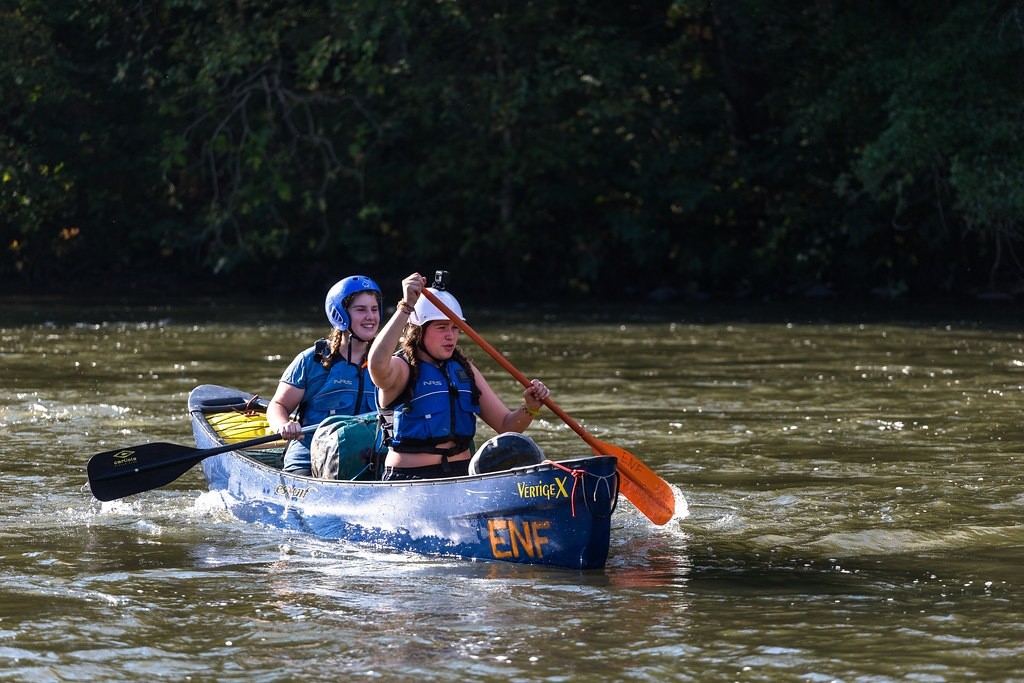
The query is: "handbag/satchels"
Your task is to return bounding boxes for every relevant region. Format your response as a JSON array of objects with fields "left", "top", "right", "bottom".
[{"left": 310, "top": 411, "right": 388, "bottom": 479}]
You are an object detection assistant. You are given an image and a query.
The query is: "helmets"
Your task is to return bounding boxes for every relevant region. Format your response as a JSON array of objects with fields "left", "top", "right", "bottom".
[
  {"left": 407, "top": 288, "right": 466, "bottom": 326},
  {"left": 325, "top": 275, "right": 383, "bottom": 331}
]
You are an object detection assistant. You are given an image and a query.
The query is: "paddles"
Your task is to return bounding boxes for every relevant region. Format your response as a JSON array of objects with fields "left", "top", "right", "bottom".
[
  {"left": 86, "top": 411, "right": 378, "bottom": 502},
  {"left": 420, "top": 284, "right": 676, "bottom": 526}
]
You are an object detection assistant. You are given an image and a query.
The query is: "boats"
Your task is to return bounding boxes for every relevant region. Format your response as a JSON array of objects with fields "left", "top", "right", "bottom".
[{"left": 185, "top": 382, "right": 621, "bottom": 573}]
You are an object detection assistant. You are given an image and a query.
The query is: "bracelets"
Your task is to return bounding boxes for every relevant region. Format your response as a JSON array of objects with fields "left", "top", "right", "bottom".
[
  {"left": 398, "top": 298, "right": 415, "bottom": 315},
  {"left": 277, "top": 428, "right": 279, "bottom": 434},
  {"left": 522, "top": 403, "right": 543, "bottom": 416}
]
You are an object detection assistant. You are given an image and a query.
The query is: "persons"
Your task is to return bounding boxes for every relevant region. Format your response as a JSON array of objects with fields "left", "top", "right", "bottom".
[
  {"left": 368, "top": 273, "right": 550, "bottom": 481},
  {"left": 265, "top": 275, "right": 383, "bottom": 478}
]
